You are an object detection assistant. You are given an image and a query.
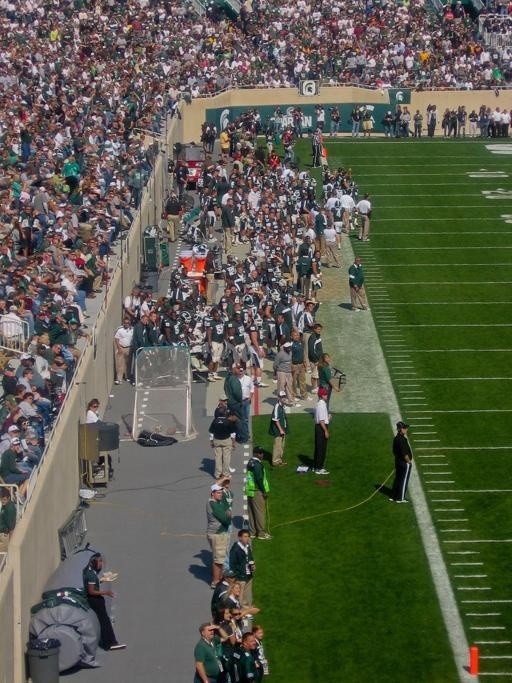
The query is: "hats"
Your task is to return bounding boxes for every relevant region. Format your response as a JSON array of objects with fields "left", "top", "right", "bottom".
[
  {"left": 278, "top": 391, "right": 287, "bottom": 398},
  {"left": 0, "top": 100, "right": 125, "bottom": 365},
  {"left": 7, "top": 424, "right": 21, "bottom": 433},
  {"left": 253, "top": 445, "right": 267, "bottom": 454},
  {"left": 167, "top": 148, "right": 370, "bottom": 268},
  {"left": 4, "top": 363, "right": 16, "bottom": 371},
  {"left": 163, "top": 269, "right": 318, "bottom": 339},
  {"left": 222, "top": 569, "right": 238, "bottom": 578},
  {"left": 11, "top": 437, "right": 22, "bottom": 445},
  {"left": 318, "top": 388, "right": 328, "bottom": 396},
  {"left": 217, "top": 394, "right": 243, "bottom": 421},
  {"left": 396, "top": 422, "right": 411, "bottom": 429},
  {"left": 17, "top": 416, "right": 28, "bottom": 426},
  {"left": 217, "top": 478, "right": 230, "bottom": 484},
  {"left": 209, "top": 485, "right": 224, "bottom": 494}
]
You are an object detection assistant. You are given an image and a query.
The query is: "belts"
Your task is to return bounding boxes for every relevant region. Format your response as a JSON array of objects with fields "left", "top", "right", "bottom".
[{"left": 207, "top": 674, "right": 217, "bottom": 678}]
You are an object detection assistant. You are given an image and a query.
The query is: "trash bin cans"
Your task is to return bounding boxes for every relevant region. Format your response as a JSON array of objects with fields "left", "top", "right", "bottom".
[
  {"left": 26, "top": 638, "right": 61, "bottom": 683},
  {"left": 140, "top": 265, "right": 159, "bottom": 293}
]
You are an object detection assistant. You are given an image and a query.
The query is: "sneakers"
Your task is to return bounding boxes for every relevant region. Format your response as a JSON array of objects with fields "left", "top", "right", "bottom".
[
  {"left": 110, "top": 643, "right": 127, "bottom": 649},
  {"left": 388, "top": 497, "right": 409, "bottom": 504}
]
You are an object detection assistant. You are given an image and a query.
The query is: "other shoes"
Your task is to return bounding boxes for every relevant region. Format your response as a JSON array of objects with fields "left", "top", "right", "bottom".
[
  {"left": 207, "top": 372, "right": 224, "bottom": 381},
  {"left": 311, "top": 468, "right": 331, "bottom": 475},
  {"left": 211, "top": 581, "right": 221, "bottom": 588},
  {"left": 350, "top": 306, "right": 360, "bottom": 313},
  {"left": 272, "top": 461, "right": 288, "bottom": 467},
  {"left": 362, "top": 306, "right": 369, "bottom": 311},
  {"left": 252, "top": 532, "right": 273, "bottom": 540},
  {"left": 288, "top": 387, "right": 319, "bottom": 407},
  {"left": 252, "top": 378, "right": 268, "bottom": 388}
]
